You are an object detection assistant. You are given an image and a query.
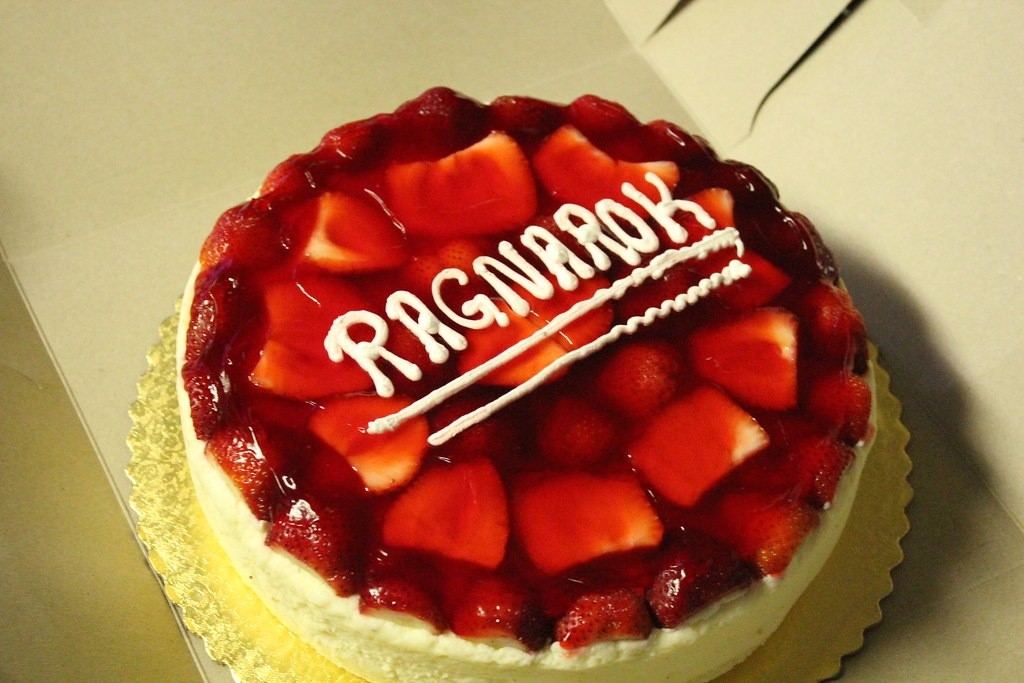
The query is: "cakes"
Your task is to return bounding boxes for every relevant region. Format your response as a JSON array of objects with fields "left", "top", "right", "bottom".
[{"left": 180, "top": 81, "right": 882, "bottom": 683}]
